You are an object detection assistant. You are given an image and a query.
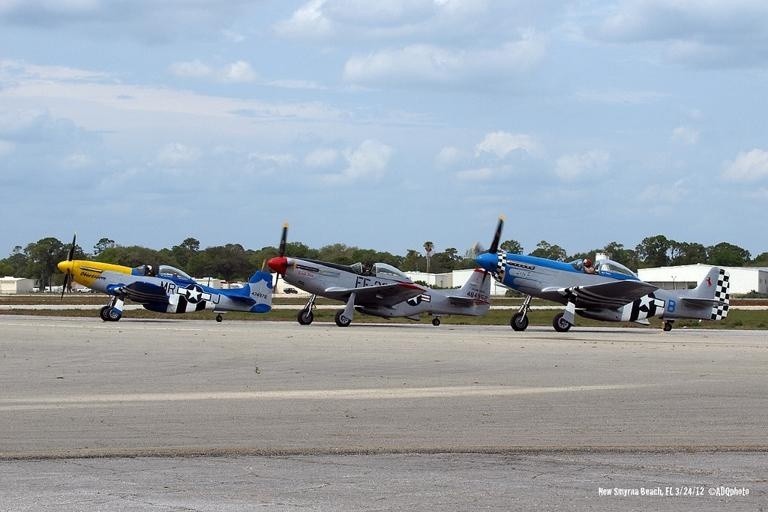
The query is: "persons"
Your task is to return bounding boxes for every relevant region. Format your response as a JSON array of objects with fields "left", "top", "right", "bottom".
[
  {"left": 362, "top": 261, "right": 375, "bottom": 277},
  {"left": 582, "top": 258, "right": 595, "bottom": 274},
  {"left": 144, "top": 264, "right": 153, "bottom": 277}
]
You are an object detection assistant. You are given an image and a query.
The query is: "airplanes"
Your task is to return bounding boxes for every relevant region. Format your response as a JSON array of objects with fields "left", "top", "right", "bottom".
[
  {"left": 58, "top": 231, "right": 274, "bottom": 323},
  {"left": 473, "top": 212, "right": 731, "bottom": 332},
  {"left": 266, "top": 219, "right": 491, "bottom": 326}
]
